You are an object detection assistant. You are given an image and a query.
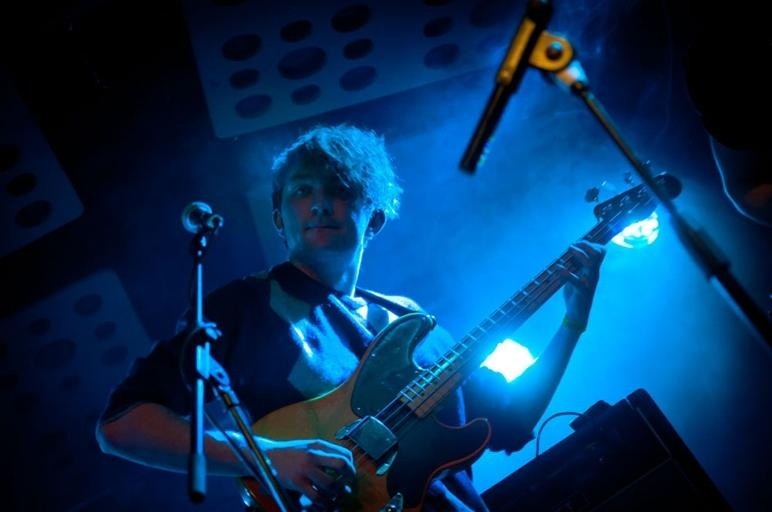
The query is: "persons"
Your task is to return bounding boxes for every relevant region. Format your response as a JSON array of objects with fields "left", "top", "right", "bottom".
[
  {"left": 685, "top": 0, "right": 771, "bottom": 226},
  {"left": 95, "top": 122, "right": 606, "bottom": 512}
]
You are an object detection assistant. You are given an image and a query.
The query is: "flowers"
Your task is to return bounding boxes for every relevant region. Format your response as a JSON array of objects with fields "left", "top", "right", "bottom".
[
  {"left": 460, "top": 0, "right": 556, "bottom": 174},
  {"left": 182, "top": 200, "right": 222, "bottom": 231}
]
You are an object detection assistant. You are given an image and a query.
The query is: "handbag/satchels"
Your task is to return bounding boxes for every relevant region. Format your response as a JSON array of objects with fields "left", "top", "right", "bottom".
[{"left": 562, "top": 314, "right": 587, "bottom": 334}]
